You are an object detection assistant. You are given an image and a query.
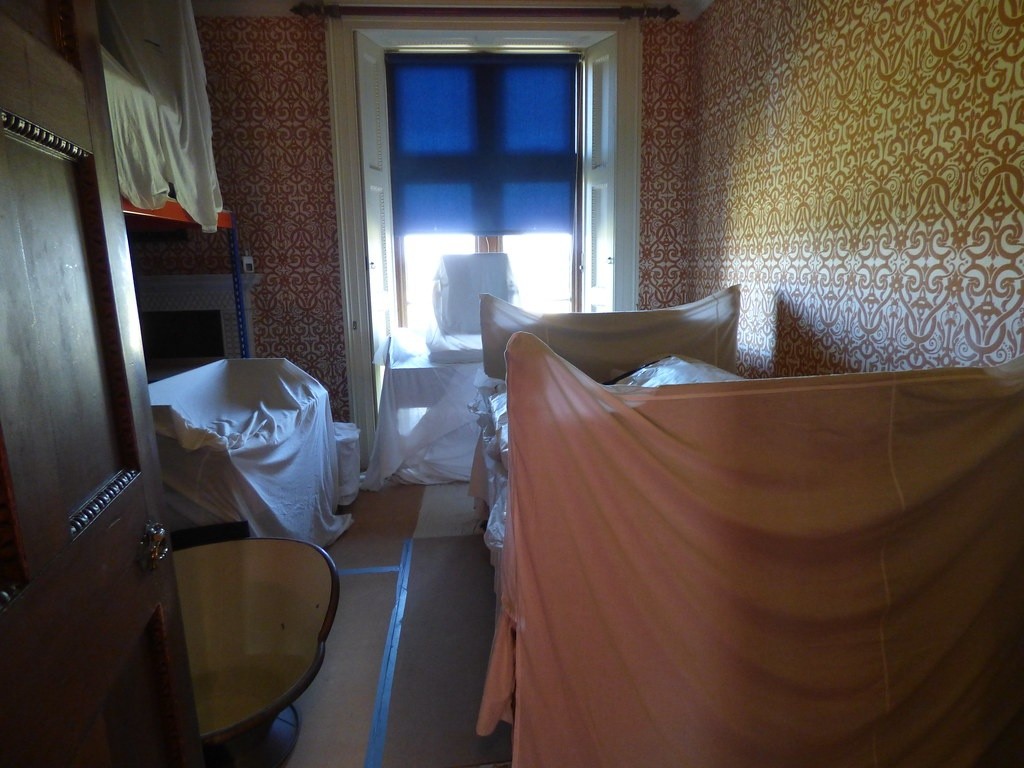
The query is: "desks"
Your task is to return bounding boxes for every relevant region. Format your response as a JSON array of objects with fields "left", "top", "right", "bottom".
[{"left": 172, "top": 538, "right": 341, "bottom": 768}]
